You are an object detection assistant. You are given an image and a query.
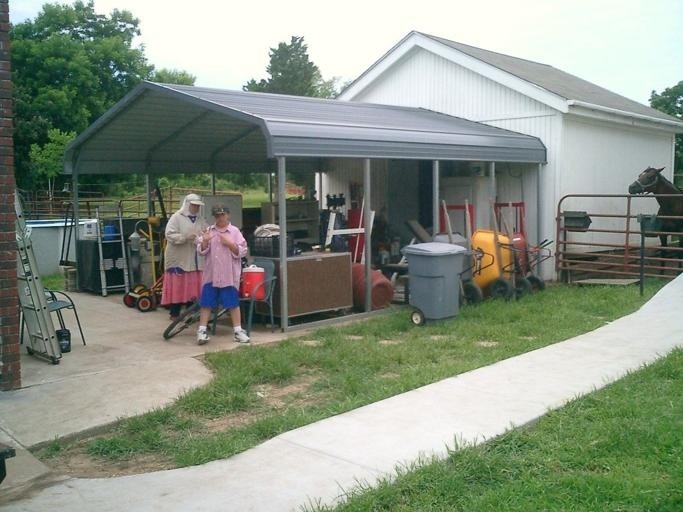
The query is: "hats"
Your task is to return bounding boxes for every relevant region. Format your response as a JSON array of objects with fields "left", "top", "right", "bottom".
[
  {"left": 210, "top": 207, "right": 230, "bottom": 214},
  {"left": 185, "top": 194, "right": 204, "bottom": 206}
]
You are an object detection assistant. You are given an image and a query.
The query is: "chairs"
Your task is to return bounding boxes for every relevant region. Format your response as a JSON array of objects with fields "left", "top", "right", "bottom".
[
  {"left": 15, "top": 274, "right": 87, "bottom": 348},
  {"left": 213, "top": 257, "right": 278, "bottom": 339}
]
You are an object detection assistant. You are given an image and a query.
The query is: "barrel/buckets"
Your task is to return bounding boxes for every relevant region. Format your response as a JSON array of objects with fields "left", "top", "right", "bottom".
[
  {"left": 352, "top": 262, "right": 394, "bottom": 309},
  {"left": 56, "top": 329, "right": 71, "bottom": 351},
  {"left": 67, "top": 269, "right": 78, "bottom": 291},
  {"left": 241, "top": 265, "right": 267, "bottom": 300},
  {"left": 84, "top": 222, "right": 98, "bottom": 239},
  {"left": 103, "top": 226, "right": 118, "bottom": 240}
]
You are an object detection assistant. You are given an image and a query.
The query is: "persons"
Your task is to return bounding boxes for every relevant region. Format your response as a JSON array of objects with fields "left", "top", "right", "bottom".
[
  {"left": 195, "top": 203, "right": 250, "bottom": 345},
  {"left": 159, "top": 193, "right": 210, "bottom": 323}
]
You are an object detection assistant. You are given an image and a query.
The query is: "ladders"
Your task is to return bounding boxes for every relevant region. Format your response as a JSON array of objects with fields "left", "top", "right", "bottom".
[
  {"left": 14, "top": 187, "right": 63, "bottom": 365},
  {"left": 96, "top": 207, "right": 131, "bottom": 296}
]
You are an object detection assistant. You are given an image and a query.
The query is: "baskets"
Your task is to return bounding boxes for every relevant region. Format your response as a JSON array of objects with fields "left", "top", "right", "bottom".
[{"left": 250, "top": 233, "right": 294, "bottom": 257}]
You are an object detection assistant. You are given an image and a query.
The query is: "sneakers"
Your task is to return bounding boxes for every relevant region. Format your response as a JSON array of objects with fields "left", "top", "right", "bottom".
[
  {"left": 196, "top": 331, "right": 210, "bottom": 345},
  {"left": 234, "top": 330, "right": 250, "bottom": 343}
]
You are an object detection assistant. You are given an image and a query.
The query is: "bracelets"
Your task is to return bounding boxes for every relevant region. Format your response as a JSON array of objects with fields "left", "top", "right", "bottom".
[{"left": 230, "top": 243, "right": 237, "bottom": 251}]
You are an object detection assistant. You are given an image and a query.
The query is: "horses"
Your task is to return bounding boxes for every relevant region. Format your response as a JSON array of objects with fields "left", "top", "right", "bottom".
[{"left": 628, "top": 166, "right": 683, "bottom": 276}]
